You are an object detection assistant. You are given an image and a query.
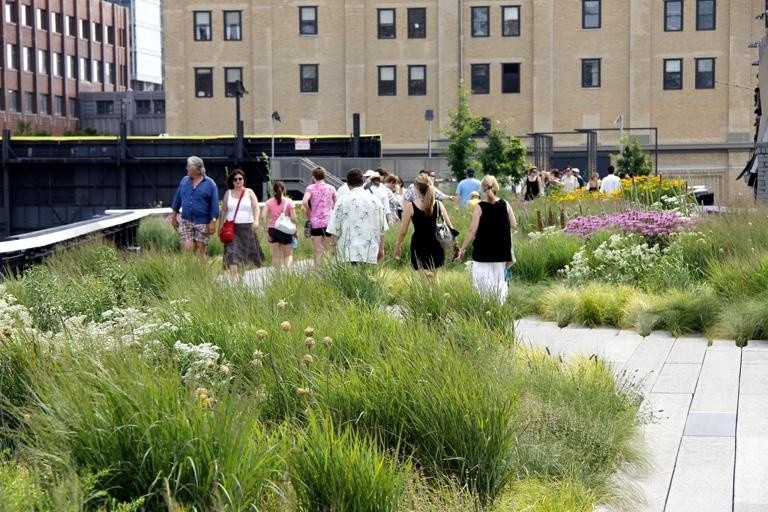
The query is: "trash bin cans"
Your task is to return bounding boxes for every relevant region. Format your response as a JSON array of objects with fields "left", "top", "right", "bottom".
[{"left": 210, "top": 217, "right": 216, "bottom": 221}]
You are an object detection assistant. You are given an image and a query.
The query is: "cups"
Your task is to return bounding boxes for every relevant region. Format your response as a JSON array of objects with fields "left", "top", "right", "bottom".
[{"left": 234, "top": 177, "right": 243, "bottom": 181}]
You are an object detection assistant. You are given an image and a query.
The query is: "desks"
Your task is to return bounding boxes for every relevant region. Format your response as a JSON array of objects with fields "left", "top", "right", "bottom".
[
  {"left": 614, "top": 114, "right": 624, "bottom": 157},
  {"left": 269, "top": 109, "right": 283, "bottom": 157},
  {"left": 222, "top": 78, "right": 251, "bottom": 168},
  {"left": 424, "top": 108, "right": 437, "bottom": 157}
]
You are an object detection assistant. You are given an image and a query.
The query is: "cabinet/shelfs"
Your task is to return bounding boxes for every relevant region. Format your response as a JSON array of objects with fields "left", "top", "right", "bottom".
[
  {"left": 305, "top": 223, "right": 312, "bottom": 238},
  {"left": 292, "top": 236, "right": 298, "bottom": 249}
]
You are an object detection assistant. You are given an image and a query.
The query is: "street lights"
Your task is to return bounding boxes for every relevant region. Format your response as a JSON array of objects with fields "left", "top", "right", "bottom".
[
  {"left": 435, "top": 219, "right": 460, "bottom": 249},
  {"left": 219, "top": 220, "right": 234, "bottom": 243},
  {"left": 304, "top": 220, "right": 312, "bottom": 238},
  {"left": 274, "top": 213, "right": 297, "bottom": 235}
]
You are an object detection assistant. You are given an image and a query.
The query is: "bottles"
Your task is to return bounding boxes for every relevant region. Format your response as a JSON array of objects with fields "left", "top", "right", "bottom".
[
  {"left": 572, "top": 168, "right": 579, "bottom": 173},
  {"left": 363, "top": 170, "right": 384, "bottom": 181}
]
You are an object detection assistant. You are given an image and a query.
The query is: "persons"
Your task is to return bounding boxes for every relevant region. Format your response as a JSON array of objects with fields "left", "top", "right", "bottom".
[
  {"left": 456, "top": 172, "right": 519, "bottom": 316},
  {"left": 219, "top": 168, "right": 261, "bottom": 287},
  {"left": 391, "top": 173, "right": 463, "bottom": 281},
  {"left": 170, "top": 155, "right": 220, "bottom": 271},
  {"left": 261, "top": 180, "right": 300, "bottom": 281}
]
